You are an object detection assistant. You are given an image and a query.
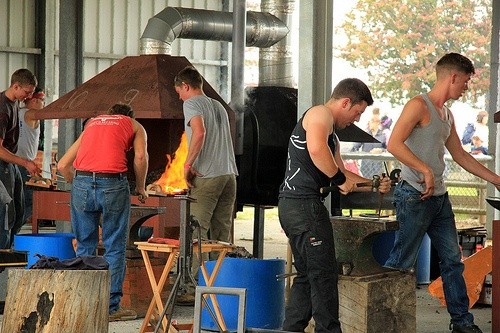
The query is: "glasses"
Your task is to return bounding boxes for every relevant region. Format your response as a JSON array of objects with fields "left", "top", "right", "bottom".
[
  {"left": 36, "top": 97, "right": 45, "bottom": 101},
  {"left": 19, "top": 86, "right": 35, "bottom": 95}
]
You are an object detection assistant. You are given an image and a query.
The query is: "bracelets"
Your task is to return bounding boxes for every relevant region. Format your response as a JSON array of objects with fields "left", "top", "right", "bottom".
[
  {"left": 184, "top": 163, "right": 191, "bottom": 169},
  {"left": 328, "top": 167, "right": 346, "bottom": 185}
]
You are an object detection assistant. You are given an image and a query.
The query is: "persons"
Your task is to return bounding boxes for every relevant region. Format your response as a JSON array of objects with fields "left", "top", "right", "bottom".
[
  {"left": 56, "top": 103, "right": 148, "bottom": 322},
  {"left": 0, "top": 69, "right": 42, "bottom": 248},
  {"left": 469, "top": 110, "right": 489, "bottom": 149},
  {"left": 277, "top": 78, "right": 392, "bottom": 333},
  {"left": 347, "top": 115, "right": 386, "bottom": 179},
  {"left": 14, "top": 88, "right": 45, "bottom": 226},
  {"left": 366, "top": 108, "right": 380, "bottom": 129},
  {"left": 171, "top": 66, "right": 240, "bottom": 305},
  {"left": 383, "top": 53, "right": 500, "bottom": 333},
  {"left": 468, "top": 131, "right": 488, "bottom": 155},
  {"left": 381, "top": 115, "right": 399, "bottom": 176}
]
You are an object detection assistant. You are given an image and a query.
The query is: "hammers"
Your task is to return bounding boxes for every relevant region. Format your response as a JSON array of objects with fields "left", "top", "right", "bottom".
[{"left": 319, "top": 175, "right": 380, "bottom": 193}]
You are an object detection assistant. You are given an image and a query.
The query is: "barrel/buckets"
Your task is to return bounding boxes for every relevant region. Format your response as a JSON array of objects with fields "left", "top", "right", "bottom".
[
  {"left": 14, "top": 233, "right": 76, "bottom": 269},
  {"left": 198, "top": 258, "right": 286, "bottom": 331}
]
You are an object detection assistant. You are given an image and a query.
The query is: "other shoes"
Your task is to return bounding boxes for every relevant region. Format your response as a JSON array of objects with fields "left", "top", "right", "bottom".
[
  {"left": 449, "top": 322, "right": 483, "bottom": 333},
  {"left": 174, "top": 292, "right": 195, "bottom": 305},
  {"left": 109, "top": 306, "right": 137, "bottom": 321}
]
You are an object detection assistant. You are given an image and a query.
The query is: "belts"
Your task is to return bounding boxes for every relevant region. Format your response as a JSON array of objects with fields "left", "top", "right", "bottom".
[{"left": 75, "top": 170, "right": 128, "bottom": 178}]
